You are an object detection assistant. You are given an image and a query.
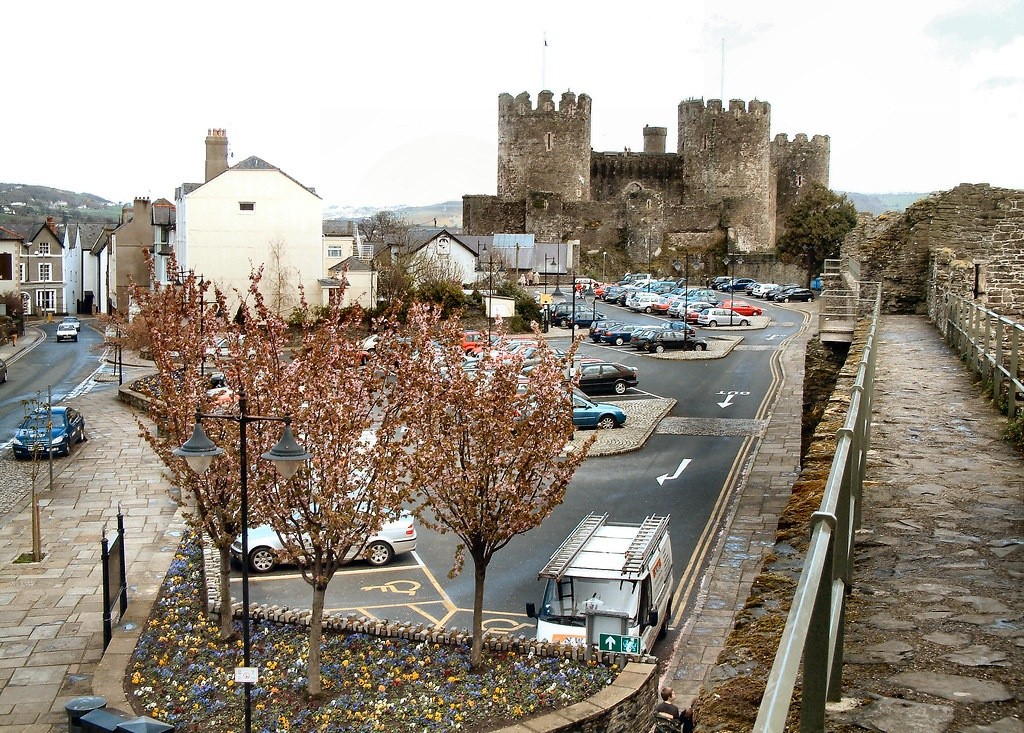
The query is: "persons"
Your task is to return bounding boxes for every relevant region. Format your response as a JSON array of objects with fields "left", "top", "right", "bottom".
[
  {"left": 520, "top": 270, "right": 540, "bottom": 286},
  {"left": 575, "top": 281, "right": 586, "bottom": 295}
]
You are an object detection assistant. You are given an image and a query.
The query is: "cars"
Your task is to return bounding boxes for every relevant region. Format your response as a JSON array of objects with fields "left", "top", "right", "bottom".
[
  {"left": 62, "top": 317, "right": 80, "bottom": 331},
  {"left": 12, "top": 405, "right": 85, "bottom": 459},
  {"left": 0, "top": 359, "right": 8, "bottom": 383},
  {"left": 140, "top": 368, "right": 232, "bottom": 400},
  {"left": 659, "top": 321, "right": 695, "bottom": 337},
  {"left": 652, "top": 298, "right": 682, "bottom": 314},
  {"left": 57, "top": 324, "right": 77, "bottom": 342},
  {"left": 817, "top": 278, "right": 835, "bottom": 287},
  {"left": 715, "top": 277, "right": 738, "bottom": 289},
  {"left": 495, "top": 338, "right": 606, "bottom": 383},
  {"left": 716, "top": 299, "right": 762, "bottom": 316},
  {"left": 680, "top": 302, "right": 715, "bottom": 319},
  {"left": 567, "top": 311, "right": 608, "bottom": 330},
  {"left": 568, "top": 362, "right": 639, "bottom": 394},
  {"left": 697, "top": 308, "right": 751, "bottom": 327},
  {"left": 719, "top": 277, "right": 757, "bottom": 292},
  {"left": 600, "top": 324, "right": 653, "bottom": 346},
  {"left": 688, "top": 295, "right": 718, "bottom": 304},
  {"left": 687, "top": 306, "right": 714, "bottom": 324},
  {"left": 622, "top": 280, "right": 693, "bottom": 299},
  {"left": 230, "top": 501, "right": 417, "bottom": 573},
  {"left": 575, "top": 279, "right": 638, "bottom": 306},
  {"left": 509, "top": 391, "right": 627, "bottom": 430},
  {"left": 360, "top": 333, "right": 531, "bottom": 406}
]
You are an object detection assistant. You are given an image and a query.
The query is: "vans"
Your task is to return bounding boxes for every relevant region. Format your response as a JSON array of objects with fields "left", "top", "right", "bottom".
[{"left": 526, "top": 510, "right": 674, "bottom": 658}]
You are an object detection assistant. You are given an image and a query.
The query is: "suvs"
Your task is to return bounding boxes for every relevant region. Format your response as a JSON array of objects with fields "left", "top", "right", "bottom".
[
  {"left": 629, "top": 295, "right": 666, "bottom": 314},
  {"left": 774, "top": 288, "right": 815, "bottom": 303},
  {"left": 744, "top": 282, "right": 764, "bottom": 296},
  {"left": 680, "top": 289, "right": 716, "bottom": 301},
  {"left": 668, "top": 301, "right": 692, "bottom": 317},
  {"left": 203, "top": 336, "right": 256, "bottom": 362},
  {"left": 616, "top": 274, "right": 652, "bottom": 286},
  {"left": 647, "top": 331, "right": 708, "bottom": 354},
  {"left": 551, "top": 302, "right": 606, "bottom": 327},
  {"left": 459, "top": 330, "right": 499, "bottom": 357},
  {"left": 752, "top": 283, "right": 782, "bottom": 298},
  {"left": 765, "top": 283, "right": 803, "bottom": 300},
  {"left": 589, "top": 319, "right": 627, "bottom": 343},
  {"left": 629, "top": 328, "right": 675, "bottom": 351}
]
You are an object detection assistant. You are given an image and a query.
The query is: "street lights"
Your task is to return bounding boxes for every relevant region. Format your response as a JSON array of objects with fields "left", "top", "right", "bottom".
[
  {"left": 370, "top": 262, "right": 377, "bottom": 310},
  {"left": 555, "top": 231, "right": 562, "bottom": 288},
  {"left": 545, "top": 253, "right": 557, "bottom": 294},
  {"left": 476, "top": 254, "right": 505, "bottom": 348},
  {"left": 722, "top": 251, "right": 745, "bottom": 327},
  {"left": 34, "top": 247, "right": 46, "bottom": 317},
  {"left": 672, "top": 248, "right": 700, "bottom": 351},
  {"left": 603, "top": 252, "right": 607, "bottom": 284},
  {"left": 572, "top": 271, "right": 595, "bottom": 343},
  {"left": 477, "top": 241, "right": 488, "bottom": 264},
  {"left": 171, "top": 385, "right": 314, "bottom": 733}
]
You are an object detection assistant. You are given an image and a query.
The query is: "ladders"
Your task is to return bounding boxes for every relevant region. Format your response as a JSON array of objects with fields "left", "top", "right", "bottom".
[
  {"left": 537, "top": 510, "right": 609, "bottom": 583},
  {"left": 621, "top": 512, "right": 671, "bottom": 577}
]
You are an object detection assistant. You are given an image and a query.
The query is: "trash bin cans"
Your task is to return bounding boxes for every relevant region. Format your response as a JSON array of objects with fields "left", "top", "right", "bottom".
[{"left": 64, "top": 696, "right": 176, "bottom": 733}]
